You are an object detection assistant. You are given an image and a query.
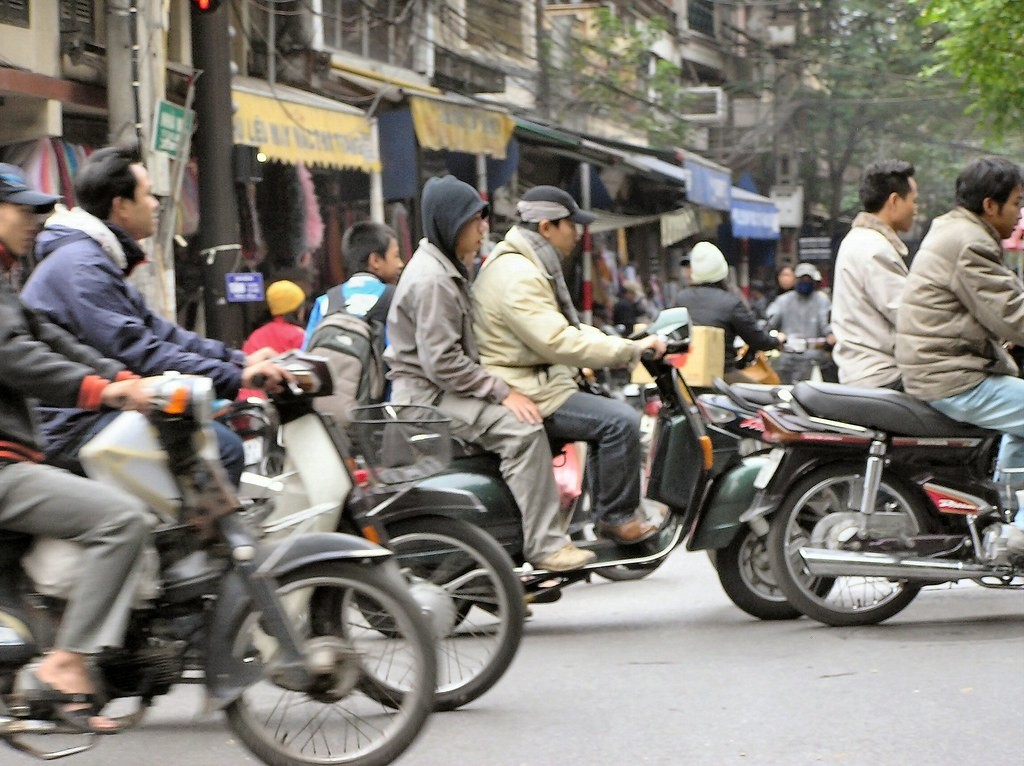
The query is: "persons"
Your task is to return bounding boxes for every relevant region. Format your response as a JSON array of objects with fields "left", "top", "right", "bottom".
[
  {"left": 896, "top": 156, "right": 1024, "bottom": 529},
  {"left": 830, "top": 157, "right": 919, "bottom": 393},
  {"left": 0, "top": 145, "right": 294, "bottom": 734},
  {"left": 612, "top": 242, "right": 839, "bottom": 386},
  {"left": 233, "top": 280, "right": 308, "bottom": 437},
  {"left": 302, "top": 175, "right": 669, "bottom": 570}
]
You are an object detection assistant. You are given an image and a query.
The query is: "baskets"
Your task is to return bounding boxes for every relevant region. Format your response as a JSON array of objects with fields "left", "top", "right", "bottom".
[
  {"left": 347, "top": 404, "right": 453, "bottom": 483},
  {"left": 772, "top": 354, "right": 812, "bottom": 382}
]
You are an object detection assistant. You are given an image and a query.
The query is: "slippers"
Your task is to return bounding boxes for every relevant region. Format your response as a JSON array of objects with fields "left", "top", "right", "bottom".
[{"left": 16, "top": 663, "right": 122, "bottom": 736}]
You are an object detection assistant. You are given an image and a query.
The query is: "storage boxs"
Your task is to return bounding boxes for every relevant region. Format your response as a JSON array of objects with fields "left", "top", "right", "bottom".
[{"left": 629, "top": 324, "right": 726, "bottom": 387}]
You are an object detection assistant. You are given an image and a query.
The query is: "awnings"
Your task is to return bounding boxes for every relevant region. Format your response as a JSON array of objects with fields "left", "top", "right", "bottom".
[{"left": 562, "top": 127, "right": 781, "bottom": 241}]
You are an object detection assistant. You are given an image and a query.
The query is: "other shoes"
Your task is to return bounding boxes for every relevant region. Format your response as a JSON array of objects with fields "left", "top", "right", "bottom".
[
  {"left": 534, "top": 546, "right": 597, "bottom": 572},
  {"left": 598, "top": 519, "right": 658, "bottom": 545},
  {"left": 982, "top": 523, "right": 1024, "bottom": 569}
]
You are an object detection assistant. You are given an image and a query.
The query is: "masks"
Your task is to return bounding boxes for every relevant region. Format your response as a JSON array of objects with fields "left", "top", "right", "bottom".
[{"left": 796, "top": 281, "right": 816, "bottom": 295}]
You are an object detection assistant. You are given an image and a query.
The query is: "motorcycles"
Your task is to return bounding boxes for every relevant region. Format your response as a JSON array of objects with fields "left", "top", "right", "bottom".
[
  {"left": 0, "top": 359, "right": 527, "bottom": 765},
  {"left": 358, "top": 326, "right": 1024, "bottom": 627}
]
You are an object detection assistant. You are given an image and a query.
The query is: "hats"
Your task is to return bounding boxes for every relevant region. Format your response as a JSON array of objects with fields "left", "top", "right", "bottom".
[
  {"left": 794, "top": 263, "right": 820, "bottom": 281},
  {"left": 690, "top": 241, "right": 729, "bottom": 284},
  {"left": 516, "top": 185, "right": 598, "bottom": 228},
  {"left": 266, "top": 280, "right": 305, "bottom": 315},
  {"left": 0, "top": 163, "right": 62, "bottom": 214}
]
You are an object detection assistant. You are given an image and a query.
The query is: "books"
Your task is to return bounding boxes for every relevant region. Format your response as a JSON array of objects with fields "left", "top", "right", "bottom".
[{"left": 178, "top": 52, "right": 623, "bottom": 172}]
